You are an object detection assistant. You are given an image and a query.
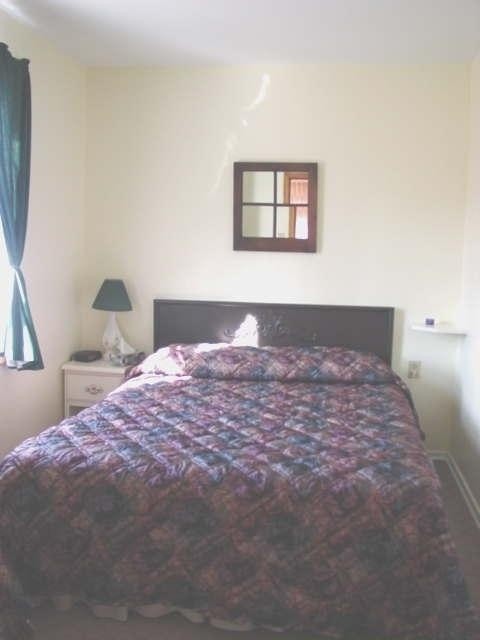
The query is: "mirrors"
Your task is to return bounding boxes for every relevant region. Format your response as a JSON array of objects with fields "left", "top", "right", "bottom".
[{"left": 232, "top": 161, "right": 318, "bottom": 254}]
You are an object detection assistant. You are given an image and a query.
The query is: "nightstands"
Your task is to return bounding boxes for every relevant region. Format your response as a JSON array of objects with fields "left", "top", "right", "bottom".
[{"left": 63, "top": 355, "right": 134, "bottom": 417}]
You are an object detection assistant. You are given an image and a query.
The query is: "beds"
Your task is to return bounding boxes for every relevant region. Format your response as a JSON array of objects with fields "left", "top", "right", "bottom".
[{"left": 1, "top": 297, "right": 479, "bottom": 639}]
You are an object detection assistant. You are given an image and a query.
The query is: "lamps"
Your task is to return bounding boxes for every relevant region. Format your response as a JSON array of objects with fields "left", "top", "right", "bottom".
[{"left": 91, "top": 278, "right": 134, "bottom": 359}]
